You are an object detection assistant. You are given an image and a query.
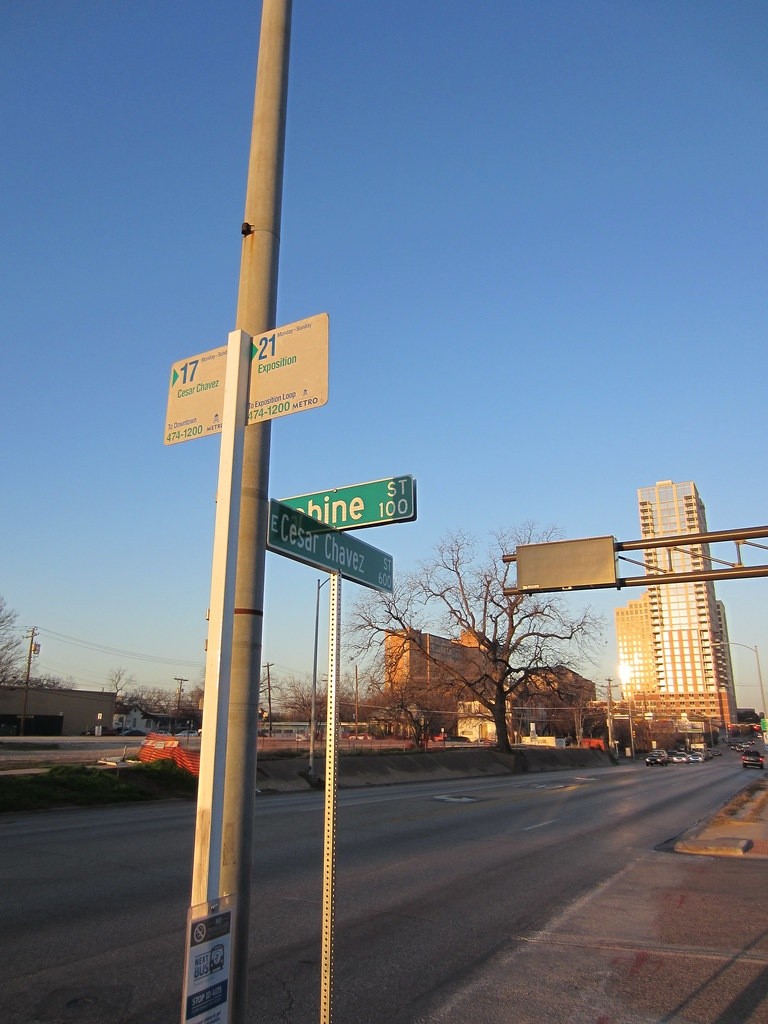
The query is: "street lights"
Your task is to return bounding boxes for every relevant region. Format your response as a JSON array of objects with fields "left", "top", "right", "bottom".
[{"left": 711, "top": 641, "right": 767, "bottom": 718}]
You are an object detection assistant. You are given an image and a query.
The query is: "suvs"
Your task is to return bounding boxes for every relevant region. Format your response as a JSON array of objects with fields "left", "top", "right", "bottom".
[{"left": 741, "top": 750, "right": 765, "bottom": 769}]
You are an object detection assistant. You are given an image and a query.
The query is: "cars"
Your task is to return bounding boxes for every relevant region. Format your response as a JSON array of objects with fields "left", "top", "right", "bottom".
[
  {"left": 644, "top": 746, "right": 721, "bottom": 767},
  {"left": 728, "top": 734, "right": 762, "bottom": 752},
  {"left": 81, "top": 724, "right": 201, "bottom": 738},
  {"left": 293, "top": 730, "right": 496, "bottom": 745}
]
